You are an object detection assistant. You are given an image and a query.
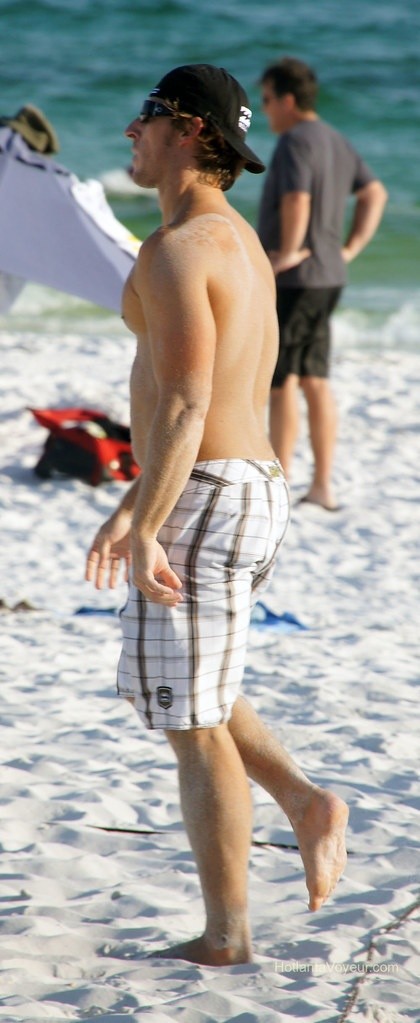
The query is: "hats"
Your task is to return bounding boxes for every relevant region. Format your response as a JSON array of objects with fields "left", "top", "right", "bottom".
[
  {"left": 1, "top": 105, "right": 59, "bottom": 155},
  {"left": 150, "top": 64, "right": 266, "bottom": 174}
]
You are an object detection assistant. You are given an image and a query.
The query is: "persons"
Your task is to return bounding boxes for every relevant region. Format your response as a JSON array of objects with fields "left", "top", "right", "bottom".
[
  {"left": 242, "top": 56, "right": 389, "bottom": 509},
  {"left": 83, "top": 61, "right": 351, "bottom": 969}
]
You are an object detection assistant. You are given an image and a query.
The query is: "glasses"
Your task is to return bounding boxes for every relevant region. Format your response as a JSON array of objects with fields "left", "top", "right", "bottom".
[
  {"left": 141, "top": 100, "right": 173, "bottom": 120},
  {"left": 261, "top": 95, "right": 276, "bottom": 106}
]
